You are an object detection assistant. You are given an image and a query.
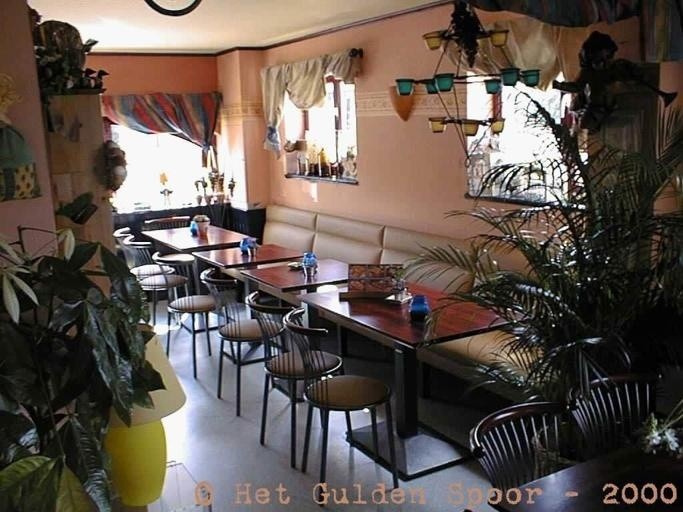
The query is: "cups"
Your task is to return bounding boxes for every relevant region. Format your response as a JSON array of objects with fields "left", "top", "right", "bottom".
[
  {"left": 304, "top": 265, "right": 316, "bottom": 277},
  {"left": 192, "top": 215, "right": 210, "bottom": 239},
  {"left": 248, "top": 248, "right": 256, "bottom": 257}
]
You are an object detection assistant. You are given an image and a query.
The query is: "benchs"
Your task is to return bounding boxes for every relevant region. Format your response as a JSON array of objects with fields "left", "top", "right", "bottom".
[{"left": 242, "top": 204, "right": 566, "bottom": 444}]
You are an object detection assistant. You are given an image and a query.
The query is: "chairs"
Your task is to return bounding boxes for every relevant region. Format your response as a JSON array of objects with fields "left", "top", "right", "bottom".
[
  {"left": 566, "top": 370, "right": 662, "bottom": 455},
  {"left": 144, "top": 215, "right": 196, "bottom": 261},
  {"left": 469, "top": 400, "right": 580, "bottom": 494},
  {"left": 112, "top": 226, "right": 399, "bottom": 507}
]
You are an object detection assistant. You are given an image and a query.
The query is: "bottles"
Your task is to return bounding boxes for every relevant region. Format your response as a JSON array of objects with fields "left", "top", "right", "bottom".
[
  {"left": 239, "top": 237, "right": 256, "bottom": 254},
  {"left": 409, "top": 294, "right": 433, "bottom": 323},
  {"left": 189, "top": 222, "right": 196, "bottom": 236},
  {"left": 320, "top": 147, "right": 328, "bottom": 176},
  {"left": 303, "top": 252, "right": 317, "bottom": 278}
]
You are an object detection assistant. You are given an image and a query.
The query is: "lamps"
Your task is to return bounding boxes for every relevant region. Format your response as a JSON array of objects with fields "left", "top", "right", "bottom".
[
  {"left": 395, "top": 1, "right": 540, "bottom": 167},
  {"left": 103, "top": 323, "right": 185, "bottom": 505}
]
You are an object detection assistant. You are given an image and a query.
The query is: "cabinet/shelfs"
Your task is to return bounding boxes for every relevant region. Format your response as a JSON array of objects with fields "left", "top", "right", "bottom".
[{"left": 42, "top": 93, "right": 116, "bottom": 320}]
